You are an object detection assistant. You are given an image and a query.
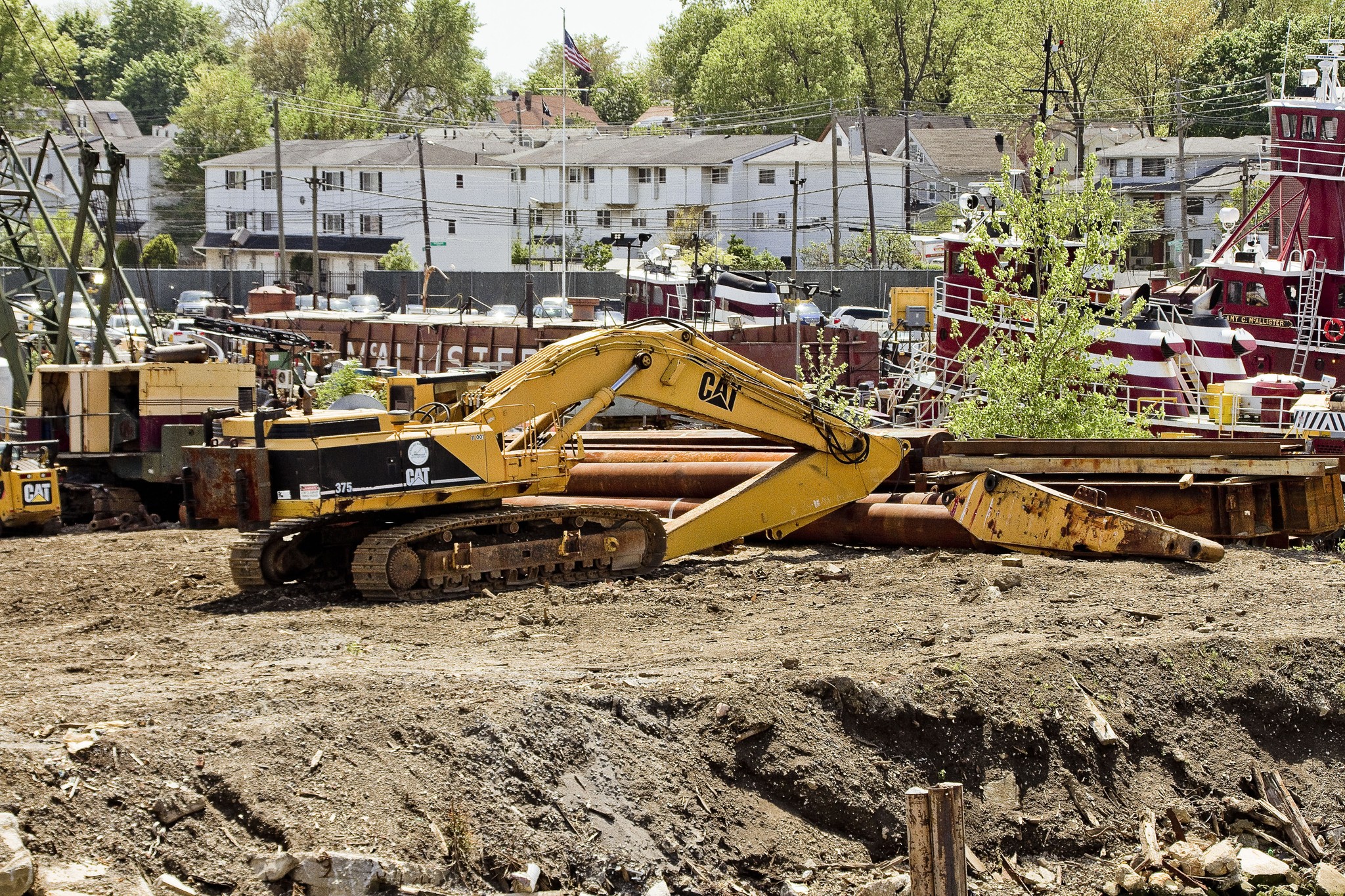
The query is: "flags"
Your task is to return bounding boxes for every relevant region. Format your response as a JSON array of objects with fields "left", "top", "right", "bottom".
[{"left": 563, "top": 29, "right": 593, "bottom": 74}]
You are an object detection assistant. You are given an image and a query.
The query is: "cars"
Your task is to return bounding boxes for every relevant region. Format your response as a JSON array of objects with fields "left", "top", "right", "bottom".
[
  {"left": 294, "top": 293, "right": 354, "bottom": 311},
  {"left": 347, "top": 294, "right": 386, "bottom": 313},
  {"left": 171, "top": 290, "right": 216, "bottom": 316},
  {"left": 488, "top": 304, "right": 519, "bottom": 319},
  {"left": 55, "top": 293, "right": 149, "bottom": 334},
  {"left": 597, "top": 311, "right": 623, "bottom": 322},
  {"left": 535, "top": 297, "right": 574, "bottom": 319},
  {"left": 786, "top": 299, "right": 828, "bottom": 327}
]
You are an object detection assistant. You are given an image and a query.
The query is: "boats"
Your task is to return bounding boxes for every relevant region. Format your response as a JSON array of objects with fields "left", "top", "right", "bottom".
[{"left": 884, "top": 11, "right": 1345, "bottom": 487}]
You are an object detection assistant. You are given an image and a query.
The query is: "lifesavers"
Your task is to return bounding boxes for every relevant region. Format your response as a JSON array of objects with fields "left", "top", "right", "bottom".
[
  {"left": 1324, "top": 318, "right": 1344, "bottom": 342},
  {"left": 1020, "top": 311, "right": 1034, "bottom": 322}
]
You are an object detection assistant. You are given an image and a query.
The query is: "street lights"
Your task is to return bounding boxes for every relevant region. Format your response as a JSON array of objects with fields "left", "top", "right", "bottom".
[{"left": 611, "top": 231, "right": 652, "bottom": 326}]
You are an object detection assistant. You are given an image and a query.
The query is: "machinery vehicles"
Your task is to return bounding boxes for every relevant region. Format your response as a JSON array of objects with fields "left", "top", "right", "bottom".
[
  {"left": 156, "top": 320, "right": 913, "bottom": 603},
  {"left": 0, "top": 438, "right": 144, "bottom": 534}
]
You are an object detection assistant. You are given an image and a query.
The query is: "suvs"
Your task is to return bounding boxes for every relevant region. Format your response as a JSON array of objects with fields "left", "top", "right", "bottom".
[{"left": 830, "top": 305, "right": 890, "bottom": 331}]
